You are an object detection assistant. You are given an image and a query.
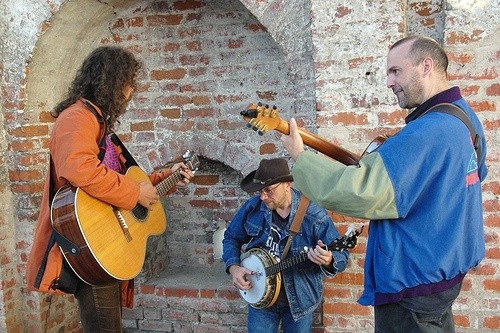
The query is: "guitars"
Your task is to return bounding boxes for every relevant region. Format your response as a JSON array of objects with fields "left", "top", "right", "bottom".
[
  {"left": 48, "top": 148, "right": 202, "bottom": 288},
  {"left": 233, "top": 223, "right": 366, "bottom": 311},
  {"left": 239, "top": 102, "right": 390, "bottom": 169}
]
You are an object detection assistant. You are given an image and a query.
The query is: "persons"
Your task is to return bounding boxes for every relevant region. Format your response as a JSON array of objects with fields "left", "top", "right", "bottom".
[
  {"left": 221, "top": 155, "right": 352, "bottom": 333},
  {"left": 26, "top": 42, "right": 198, "bottom": 333},
  {"left": 280, "top": 35, "right": 488, "bottom": 333}
]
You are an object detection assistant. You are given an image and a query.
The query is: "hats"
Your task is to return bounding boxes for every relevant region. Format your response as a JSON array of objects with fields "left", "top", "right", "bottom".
[{"left": 240, "top": 158, "right": 294, "bottom": 193}]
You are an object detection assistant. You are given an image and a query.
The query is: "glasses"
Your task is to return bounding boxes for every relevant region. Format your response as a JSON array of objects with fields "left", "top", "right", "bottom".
[{"left": 254, "top": 182, "right": 282, "bottom": 196}]
